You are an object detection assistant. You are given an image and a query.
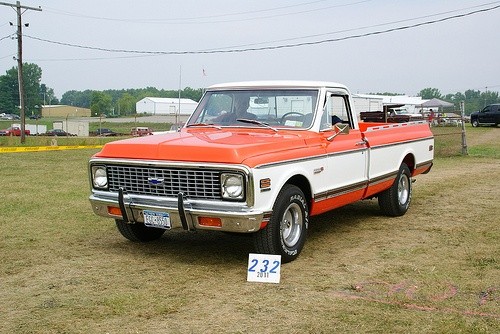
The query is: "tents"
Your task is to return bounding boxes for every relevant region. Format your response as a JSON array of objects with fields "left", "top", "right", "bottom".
[{"left": 415, "top": 98, "right": 454, "bottom": 115}]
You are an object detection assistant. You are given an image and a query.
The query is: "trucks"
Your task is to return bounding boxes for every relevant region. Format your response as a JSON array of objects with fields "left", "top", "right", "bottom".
[
  {"left": 130, "top": 127, "right": 154, "bottom": 137},
  {"left": 359, "top": 103, "right": 423, "bottom": 121}
]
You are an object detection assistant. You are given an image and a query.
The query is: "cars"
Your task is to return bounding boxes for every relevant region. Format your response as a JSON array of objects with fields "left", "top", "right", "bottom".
[
  {"left": 93, "top": 126, "right": 114, "bottom": 136},
  {"left": 468, "top": 102, "right": 497, "bottom": 128},
  {"left": 2, "top": 112, "right": 21, "bottom": 121},
  {"left": 30, "top": 112, "right": 43, "bottom": 119},
  {"left": 47, "top": 128, "right": 72, "bottom": 137}
]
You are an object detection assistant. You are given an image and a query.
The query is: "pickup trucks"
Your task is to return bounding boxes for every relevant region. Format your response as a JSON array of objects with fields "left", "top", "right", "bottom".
[
  {"left": 2, "top": 125, "right": 31, "bottom": 137},
  {"left": 87, "top": 79, "right": 436, "bottom": 263}
]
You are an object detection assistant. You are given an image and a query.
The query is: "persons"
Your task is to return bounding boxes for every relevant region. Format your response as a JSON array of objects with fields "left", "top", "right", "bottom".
[
  {"left": 209, "top": 94, "right": 257, "bottom": 126},
  {"left": 419, "top": 108, "right": 448, "bottom": 127},
  {"left": 389, "top": 109, "right": 396, "bottom": 117},
  {"left": 302, "top": 92, "right": 343, "bottom": 127}
]
